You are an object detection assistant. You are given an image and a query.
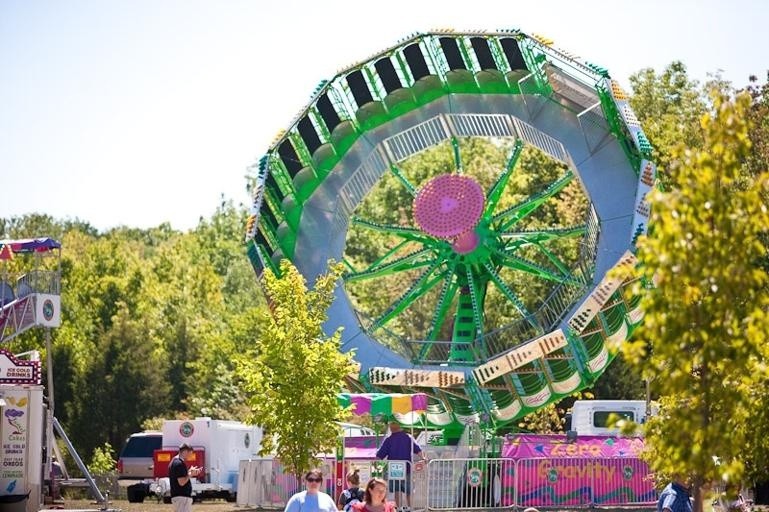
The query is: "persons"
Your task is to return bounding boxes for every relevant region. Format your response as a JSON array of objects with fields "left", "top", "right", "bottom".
[
  {"left": 338, "top": 469, "right": 367, "bottom": 510},
  {"left": 377, "top": 422, "right": 426, "bottom": 512},
  {"left": 657, "top": 473, "right": 694, "bottom": 512},
  {"left": 168, "top": 443, "right": 203, "bottom": 512},
  {"left": 717, "top": 482, "right": 748, "bottom": 512},
  {"left": 50, "top": 455, "right": 65, "bottom": 502},
  {"left": 284, "top": 470, "right": 339, "bottom": 512},
  {"left": 352, "top": 479, "right": 397, "bottom": 512}
]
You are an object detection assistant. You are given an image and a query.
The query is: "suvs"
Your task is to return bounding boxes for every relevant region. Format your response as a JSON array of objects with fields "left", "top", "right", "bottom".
[{"left": 116, "top": 430, "right": 164, "bottom": 503}]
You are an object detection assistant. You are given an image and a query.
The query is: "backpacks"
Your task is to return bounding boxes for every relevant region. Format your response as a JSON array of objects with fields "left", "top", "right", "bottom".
[{"left": 342, "top": 488, "right": 362, "bottom": 512}]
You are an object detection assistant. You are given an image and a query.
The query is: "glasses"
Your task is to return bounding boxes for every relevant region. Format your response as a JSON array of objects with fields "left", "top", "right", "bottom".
[{"left": 306, "top": 478, "right": 321, "bottom": 483}]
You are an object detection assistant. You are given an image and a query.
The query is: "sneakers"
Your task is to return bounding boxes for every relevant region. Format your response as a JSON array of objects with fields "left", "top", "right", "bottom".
[{"left": 394, "top": 505, "right": 410, "bottom": 512}]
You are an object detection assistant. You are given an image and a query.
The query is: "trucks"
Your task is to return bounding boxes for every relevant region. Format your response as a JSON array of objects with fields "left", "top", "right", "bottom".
[{"left": 149, "top": 416, "right": 269, "bottom": 504}]
